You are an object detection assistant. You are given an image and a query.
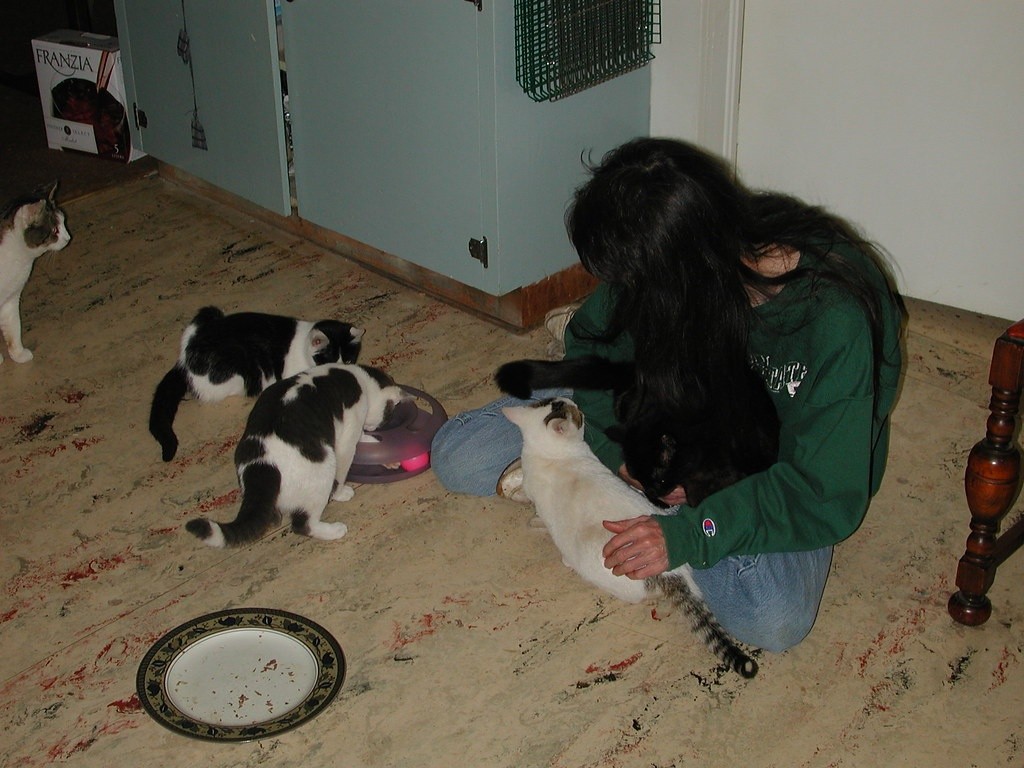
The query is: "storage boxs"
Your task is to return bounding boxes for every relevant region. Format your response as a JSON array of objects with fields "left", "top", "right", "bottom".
[{"left": 31, "top": 29, "right": 147, "bottom": 164}]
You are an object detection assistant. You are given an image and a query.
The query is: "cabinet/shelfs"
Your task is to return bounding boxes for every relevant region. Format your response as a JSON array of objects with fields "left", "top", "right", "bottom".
[{"left": 114, "top": 0, "right": 650, "bottom": 335}]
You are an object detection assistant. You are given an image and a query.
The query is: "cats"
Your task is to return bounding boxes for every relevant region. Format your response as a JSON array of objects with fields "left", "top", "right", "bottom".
[
  {"left": 185, "top": 362, "right": 413, "bottom": 548},
  {"left": 502, "top": 396, "right": 759, "bottom": 680},
  {"left": 0, "top": 179, "right": 70, "bottom": 364},
  {"left": 148, "top": 304, "right": 366, "bottom": 463}
]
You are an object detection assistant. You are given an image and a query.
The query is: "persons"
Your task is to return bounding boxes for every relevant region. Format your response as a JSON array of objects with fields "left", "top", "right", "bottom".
[{"left": 429, "top": 137, "right": 902, "bottom": 654}]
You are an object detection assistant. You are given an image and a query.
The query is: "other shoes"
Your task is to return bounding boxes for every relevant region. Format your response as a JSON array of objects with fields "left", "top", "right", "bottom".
[
  {"left": 544, "top": 303, "right": 582, "bottom": 343},
  {"left": 496, "top": 457, "right": 532, "bottom": 504}
]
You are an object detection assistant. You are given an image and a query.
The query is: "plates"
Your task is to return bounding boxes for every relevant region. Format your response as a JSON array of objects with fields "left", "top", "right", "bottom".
[{"left": 136, "top": 606, "right": 345, "bottom": 739}]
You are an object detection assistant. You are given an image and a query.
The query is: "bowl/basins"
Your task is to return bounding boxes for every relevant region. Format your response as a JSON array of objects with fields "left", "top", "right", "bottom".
[{"left": 334, "top": 380, "right": 449, "bottom": 483}]
